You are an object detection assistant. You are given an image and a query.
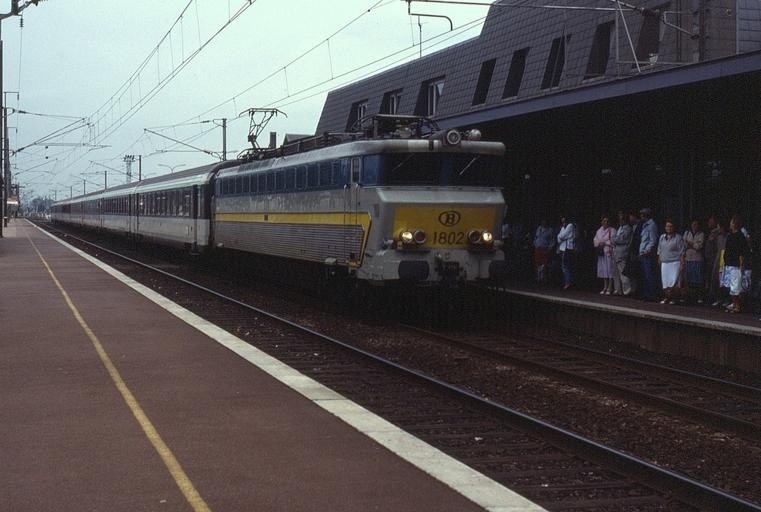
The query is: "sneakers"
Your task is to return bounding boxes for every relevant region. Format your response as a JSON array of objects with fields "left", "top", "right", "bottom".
[{"left": 530, "top": 281, "right": 744, "bottom": 315}]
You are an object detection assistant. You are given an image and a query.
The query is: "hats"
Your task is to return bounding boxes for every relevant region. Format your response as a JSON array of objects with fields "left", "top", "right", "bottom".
[{"left": 639, "top": 208, "right": 651, "bottom": 217}]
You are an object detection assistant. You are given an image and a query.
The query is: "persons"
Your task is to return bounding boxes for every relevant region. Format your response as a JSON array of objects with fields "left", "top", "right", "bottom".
[{"left": 502, "top": 205, "right": 761, "bottom": 316}]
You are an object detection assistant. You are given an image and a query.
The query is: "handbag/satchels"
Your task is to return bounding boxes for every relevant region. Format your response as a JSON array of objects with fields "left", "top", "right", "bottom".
[
  {"left": 666, "top": 270, "right": 688, "bottom": 300},
  {"left": 563, "top": 238, "right": 576, "bottom": 267},
  {"left": 594, "top": 243, "right": 603, "bottom": 257}
]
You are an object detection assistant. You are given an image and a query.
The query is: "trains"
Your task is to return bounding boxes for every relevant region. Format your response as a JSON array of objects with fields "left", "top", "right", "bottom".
[{"left": 48, "top": 104, "right": 513, "bottom": 311}]
